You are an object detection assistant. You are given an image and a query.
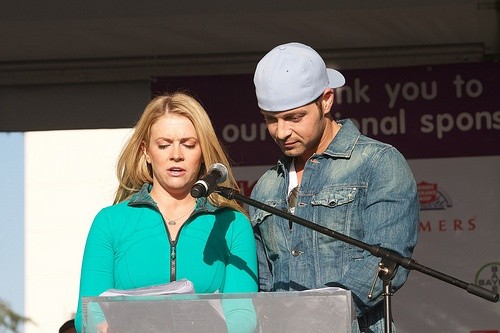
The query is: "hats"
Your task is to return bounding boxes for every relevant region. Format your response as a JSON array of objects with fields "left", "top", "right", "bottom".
[{"left": 253, "top": 42, "right": 345, "bottom": 111}]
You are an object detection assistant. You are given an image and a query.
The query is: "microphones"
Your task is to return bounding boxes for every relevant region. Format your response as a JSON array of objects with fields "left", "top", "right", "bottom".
[{"left": 190, "top": 163, "right": 228, "bottom": 198}]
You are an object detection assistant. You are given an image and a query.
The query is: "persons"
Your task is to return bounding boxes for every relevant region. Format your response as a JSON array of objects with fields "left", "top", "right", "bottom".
[
  {"left": 74, "top": 92, "right": 257, "bottom": 333},
  {"left": 249, "top": 41, "right": 420, "bottom": 333}
]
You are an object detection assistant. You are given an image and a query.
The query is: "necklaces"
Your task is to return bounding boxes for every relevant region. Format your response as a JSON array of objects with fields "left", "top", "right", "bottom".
[{"left": 161, "top": 203, "right": 196, "bottom": 225}]
